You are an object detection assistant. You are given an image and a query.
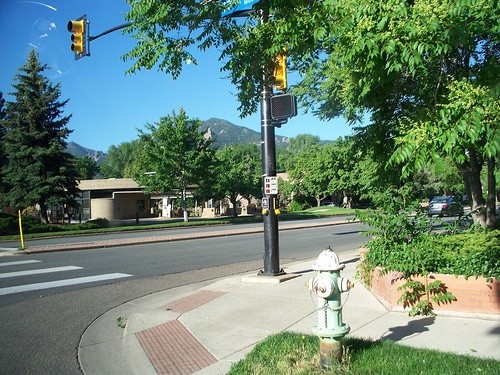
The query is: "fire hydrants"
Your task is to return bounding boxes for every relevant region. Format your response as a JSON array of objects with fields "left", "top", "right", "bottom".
[{"left": 306, "top": 245, "right": 354, "bottom": 368}]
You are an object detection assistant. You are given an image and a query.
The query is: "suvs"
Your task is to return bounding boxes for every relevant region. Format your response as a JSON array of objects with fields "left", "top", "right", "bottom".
[{"left": 428, "top": 196, "right": 464, "bottom": 216}]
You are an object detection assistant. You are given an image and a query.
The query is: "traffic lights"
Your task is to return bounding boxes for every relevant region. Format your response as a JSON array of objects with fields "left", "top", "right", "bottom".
[
  {"left": 67, "top": 14, "right": 90, "bottom": 60},
  {"left": 270, "top": 93, "right": 297, "bottom": 119},
  {"left": 273, "top": 45, "right": 286, "bottom": 89}
]
